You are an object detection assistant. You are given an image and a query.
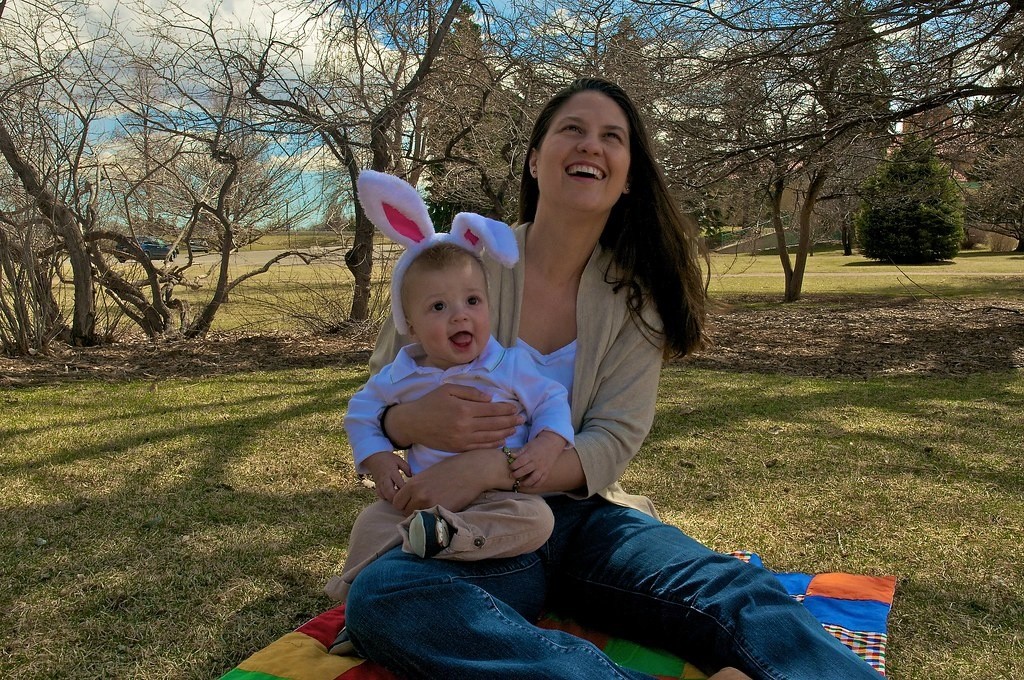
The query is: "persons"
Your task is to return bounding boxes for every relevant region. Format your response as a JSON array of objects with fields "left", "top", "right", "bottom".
[
  {"left": 345, "top": 80, "right": 889, "bottom": 680},
  {"left": 326, "top": 169, "right": 576, "bottom": 656}
]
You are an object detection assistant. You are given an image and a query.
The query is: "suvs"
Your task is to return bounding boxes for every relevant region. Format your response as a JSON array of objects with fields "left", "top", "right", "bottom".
[
  {"left": 114, "top": 235, "right": 177, "bottom": 263},
  {"left": 189, "top": 237, "right": 208, "bottom": 253}
]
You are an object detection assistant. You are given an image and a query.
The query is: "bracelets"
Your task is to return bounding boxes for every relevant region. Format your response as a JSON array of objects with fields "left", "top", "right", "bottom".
[{"left": 503, "top": 446, "right": 521, "bottom": 490}]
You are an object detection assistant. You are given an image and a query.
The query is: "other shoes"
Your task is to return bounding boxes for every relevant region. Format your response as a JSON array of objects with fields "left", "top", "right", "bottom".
[
  {"left": 327, "top": 624, "right": 355, "bottom": 656},
  {"left": 409, "top": 512, "right": 450, "bottom": 560}
]
